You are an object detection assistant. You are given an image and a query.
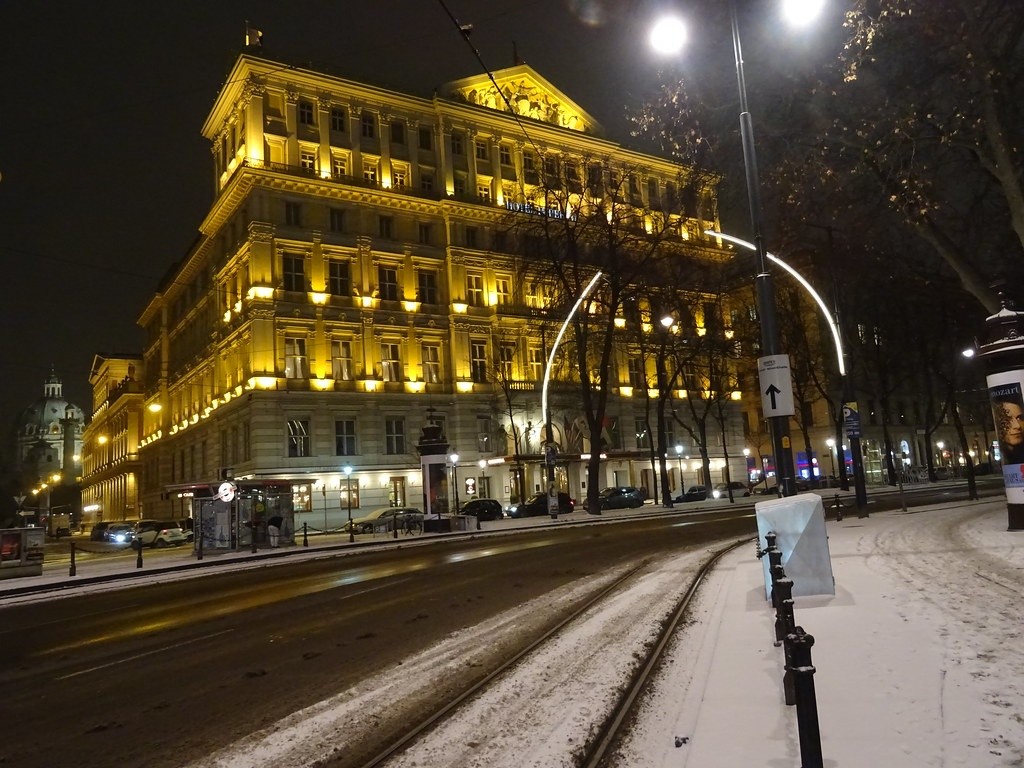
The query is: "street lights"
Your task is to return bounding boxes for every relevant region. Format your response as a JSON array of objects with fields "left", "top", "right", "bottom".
[
  {"left": 743, "top": 446, "right": 752, "bottom": 493},
  {"left": 647, "top": 1, "right": 828, "bottom": 499},
  {"left": 674, "top": 440, "right": 685, "bottom": 495},
  {"left": 825, "top": 438, "right": 836, "bottom": 481},
  {"left": 449, "top": 447, "right": 460, "bottom": 516},
  {"left": 936, "top": 439, "right": 944, "bottom": 466},
  {"left": 478, "top": 455, "right": 486, "bottom": 498},
  {"left": 972, "top": 432, "right": 981, "bottom": 464},
  {"left": 343, "top": 461, "right": 353, "bottom": 520}
]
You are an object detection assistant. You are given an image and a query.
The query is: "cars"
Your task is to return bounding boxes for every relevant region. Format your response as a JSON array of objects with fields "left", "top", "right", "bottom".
[
  {"left": 454, "top": 498, "right": 505, "bottom": 522},
  {"left": 504, "top": 491, "right": 576, "bottom": 519},
  {"left": 794, "top": 473, "right": 856, "bottom": 492},
  {"left": 675, "top": 484, "right": 706, "bottom": 503},
  {"left": 917, "top": 466, "right": 952, "bottom": 483},
  {"left": 712, "top": 481, "right": 751, "bottom": 499},
  {"left": 90, "top": 519, "right": 194, "bottom": 548},
  {"left": 583, "top": 486, "right": 644, "bottom": 511},
  {"left": 345, "top": 506, "right": 425, "bottom": 534}
]
